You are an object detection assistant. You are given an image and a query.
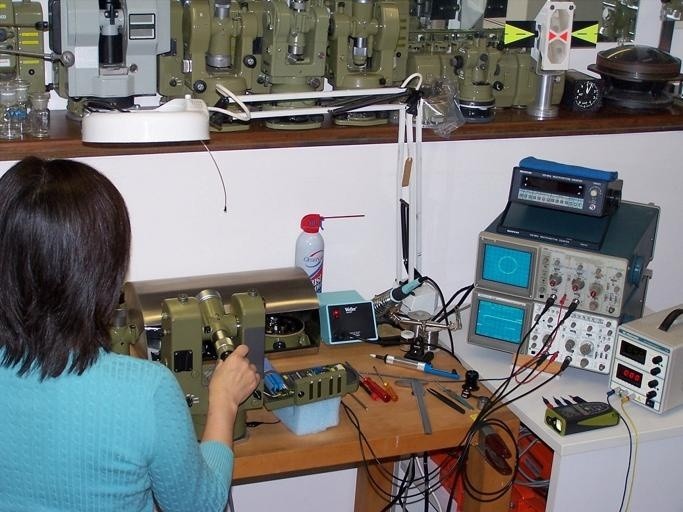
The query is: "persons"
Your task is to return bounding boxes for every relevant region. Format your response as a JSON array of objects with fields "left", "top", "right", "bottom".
[{"left": 0, "top": 156, "right": 260, "bottom": 511}]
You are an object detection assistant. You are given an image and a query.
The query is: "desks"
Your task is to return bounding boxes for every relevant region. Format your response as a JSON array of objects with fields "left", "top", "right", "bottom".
[{"left": 128, "top": 310, "right": 520, "bottom": 512}]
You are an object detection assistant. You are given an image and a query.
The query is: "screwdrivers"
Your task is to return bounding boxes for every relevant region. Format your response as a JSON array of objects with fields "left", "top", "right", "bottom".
[{"left": 345, "top": 360, "right": 398, "bottom": 402}]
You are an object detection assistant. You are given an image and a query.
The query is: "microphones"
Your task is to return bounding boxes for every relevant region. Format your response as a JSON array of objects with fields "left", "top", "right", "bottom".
[{"left": 371, "top": 276, "right": 427, "bottom": 318}]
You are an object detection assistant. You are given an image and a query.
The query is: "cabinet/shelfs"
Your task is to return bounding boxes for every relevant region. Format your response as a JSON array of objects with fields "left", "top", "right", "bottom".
[{"left": 392, "top": 305, "right": 683, "bottom": 512}]
